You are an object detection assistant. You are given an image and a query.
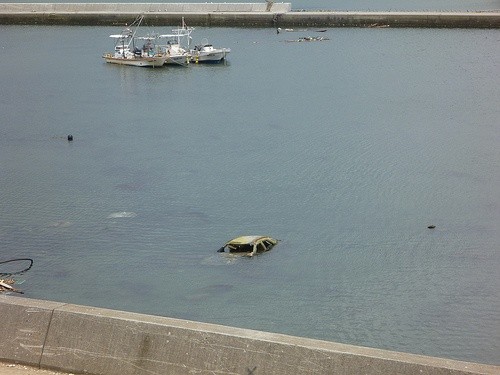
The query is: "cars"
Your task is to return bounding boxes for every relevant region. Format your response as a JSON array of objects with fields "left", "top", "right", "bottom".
[{"left": 217, "top": 235, "right": 280, "bottom": 255}]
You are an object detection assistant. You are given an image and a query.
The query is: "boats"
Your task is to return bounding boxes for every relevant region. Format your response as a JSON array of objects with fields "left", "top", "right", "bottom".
[{"left": 101, "top": 12, "right": 232, "bottom": 68}]
